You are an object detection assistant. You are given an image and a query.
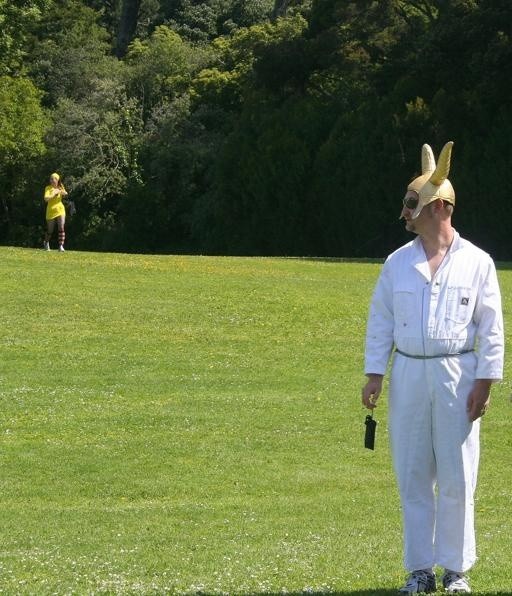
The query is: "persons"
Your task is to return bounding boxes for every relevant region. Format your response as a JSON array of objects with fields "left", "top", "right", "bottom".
[
  {"left": 361, "top": 171, "right": 504, "bottom": 595},
  {"left": 43, "top": 172, "right": 68, "bottom": 252}
]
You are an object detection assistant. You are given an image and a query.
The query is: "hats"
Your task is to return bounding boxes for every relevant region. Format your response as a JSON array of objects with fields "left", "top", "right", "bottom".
[
  {"left": 398, "top": 141, "right": 456, "bottom": 221},
  {"left": 50, "top": 172, "right": 60, "bottom": 181}
]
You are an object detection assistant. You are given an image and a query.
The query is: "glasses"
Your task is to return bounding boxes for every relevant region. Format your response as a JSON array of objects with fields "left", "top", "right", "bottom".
[{"left": 401, "top": 197, "right": 419, "bottom": 209}]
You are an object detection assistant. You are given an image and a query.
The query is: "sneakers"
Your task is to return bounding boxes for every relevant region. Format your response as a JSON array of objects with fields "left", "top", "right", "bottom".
[
  {"left": 59, "top": 245, "right": 65, "bottom": 252},
  {"left": 396, "top": 570, "right": 436, "bottom": 594},
  {"left": 44, "top": 240, "right": 50, "bottom": 251},
  {"left": 442, "top": 573, "right": 471, "bottom": 594}
]
